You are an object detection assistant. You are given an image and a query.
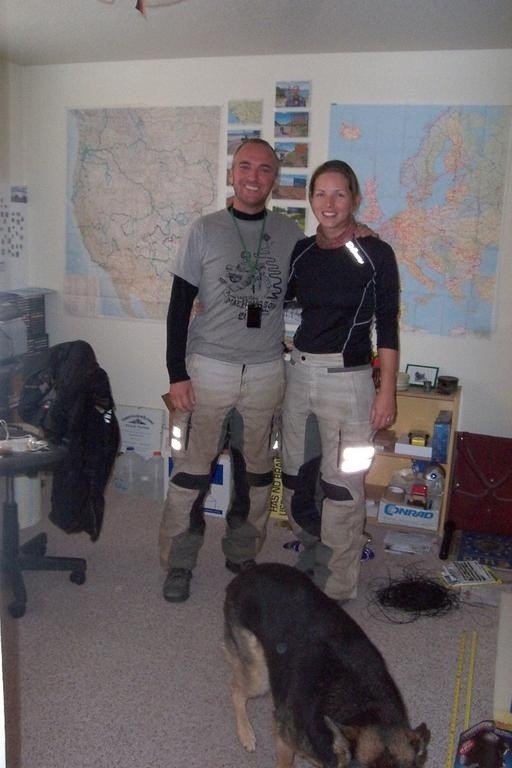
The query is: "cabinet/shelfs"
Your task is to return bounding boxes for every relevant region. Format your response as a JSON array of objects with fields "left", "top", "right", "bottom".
[{"left": 361, "top": 369, "right": 463, "bottom": 560}]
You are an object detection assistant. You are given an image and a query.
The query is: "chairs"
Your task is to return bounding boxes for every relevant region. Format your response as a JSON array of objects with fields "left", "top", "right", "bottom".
[{"left": 0, "top": 337, "right": 101, "bottom": 618}]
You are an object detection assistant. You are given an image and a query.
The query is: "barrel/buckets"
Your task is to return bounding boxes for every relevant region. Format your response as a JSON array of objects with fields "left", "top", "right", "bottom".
[
  {"left": 112, "top": 446, "right": 146, "bottom": 495},
  {"left": 143, "top": 451, "right": 165, "bottom": 504}
]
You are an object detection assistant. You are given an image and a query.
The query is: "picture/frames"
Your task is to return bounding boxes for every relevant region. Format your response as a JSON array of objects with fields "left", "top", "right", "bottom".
[{"left": 406, "top": 363, "right": 440, "bottom": 388}]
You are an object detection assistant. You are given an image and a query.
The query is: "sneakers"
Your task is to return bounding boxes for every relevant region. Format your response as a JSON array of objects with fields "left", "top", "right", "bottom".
[
  {"left": 163, "top": 569, "right": 191, "bottom": 602},
  {"left": 225, "top": 559, "right": 257, "bottom": 574}
]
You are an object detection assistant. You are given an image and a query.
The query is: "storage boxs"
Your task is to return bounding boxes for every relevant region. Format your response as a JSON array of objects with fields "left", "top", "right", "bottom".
[
  {"left": 163, "top": 446, "right": 235, "bottom": 519},
  {"left": 159, "top": 389, "right": 177, "bottom": 447}
]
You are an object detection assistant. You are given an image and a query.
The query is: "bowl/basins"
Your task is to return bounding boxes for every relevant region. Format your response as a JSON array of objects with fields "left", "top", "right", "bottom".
[
  {"left": 395, "top": 372, "right": 411, "bottom": 392},
  {"left": 435, "top": 375, "right": 458, "bottom": 396}
]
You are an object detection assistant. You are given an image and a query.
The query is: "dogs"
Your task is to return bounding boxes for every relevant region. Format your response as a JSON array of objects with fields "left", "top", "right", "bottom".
[{"left": 219, "top": 561, "right": 432, "bottom": 768}]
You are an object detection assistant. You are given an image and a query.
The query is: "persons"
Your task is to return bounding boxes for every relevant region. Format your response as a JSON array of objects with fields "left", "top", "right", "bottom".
[
  {"left": 158, "top": 140, "right": 379, "bottom": 603},
  {"left": 194, "top": 160, "right": 398, "bottom": 605}
]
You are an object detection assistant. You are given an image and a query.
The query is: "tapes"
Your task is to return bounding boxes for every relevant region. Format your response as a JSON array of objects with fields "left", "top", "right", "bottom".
[{"left": 385, "top": 485, "right": 406, "bottom": 504}]
